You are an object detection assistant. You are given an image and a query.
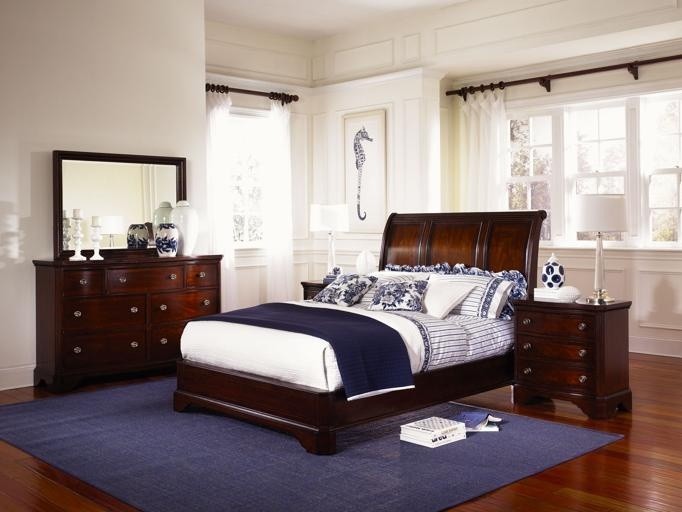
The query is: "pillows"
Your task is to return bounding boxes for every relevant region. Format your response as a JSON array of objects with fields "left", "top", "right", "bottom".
[{"left": 312, "top": 264, "right": 524, "bottom": 319}]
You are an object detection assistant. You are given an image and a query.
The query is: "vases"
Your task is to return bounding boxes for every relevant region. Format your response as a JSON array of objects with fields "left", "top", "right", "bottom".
[
  {"left": 173, "top": 199, "right": 195, "bottom": 257},
  {"left": 128, "top": 221, "right": 148, "bottom": 249},
  {"left": 154, "top": 222, "right": 180, "bottom": 259}
]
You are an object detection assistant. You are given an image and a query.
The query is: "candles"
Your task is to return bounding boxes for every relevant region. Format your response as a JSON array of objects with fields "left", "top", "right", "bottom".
[
  {"left": 66, "top": 217, "right": 71, "bottom": 227},
  {"left": 64, "top": 208, "right": 69, "bottom": 219},
  {"left": 90, "top": 217, "right": 103, "bottom": 227},
  {"left": 72, "top": 208, "right": 83, "bottom": 220}
]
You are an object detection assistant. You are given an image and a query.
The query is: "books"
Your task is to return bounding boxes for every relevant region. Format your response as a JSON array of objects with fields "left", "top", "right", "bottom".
[{"left": 400, "top": 411, "right": 503, "bottom": 449}]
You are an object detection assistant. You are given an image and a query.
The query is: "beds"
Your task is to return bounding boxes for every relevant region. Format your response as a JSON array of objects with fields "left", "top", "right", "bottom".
[{"left": 170, "top": 207, "right": 547, "bottom": 457}]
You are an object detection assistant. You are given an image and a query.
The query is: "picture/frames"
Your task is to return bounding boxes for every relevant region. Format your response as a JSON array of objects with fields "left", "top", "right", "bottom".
[{"left": 339, "top": 108, "right": 388, "bottom": 222}]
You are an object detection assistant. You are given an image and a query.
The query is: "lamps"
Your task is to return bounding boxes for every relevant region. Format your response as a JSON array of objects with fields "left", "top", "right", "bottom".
[
  {"left": 308, "top": 201, "right": 350, "bottom": 279},
  {"left": 572, "top": 192, "right": 629, "bottom": 303},
  {"left": 99, "top": 214, "right": 126, "bottom": 249}
]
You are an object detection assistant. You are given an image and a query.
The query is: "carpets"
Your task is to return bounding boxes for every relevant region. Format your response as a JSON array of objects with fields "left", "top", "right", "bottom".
[{"left": 1, "top": 365, "right": 629, "bottom": 512}]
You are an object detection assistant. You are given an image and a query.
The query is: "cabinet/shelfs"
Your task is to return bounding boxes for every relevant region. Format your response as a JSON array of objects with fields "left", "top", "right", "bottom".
[{"left": 30, "top": 250, "right": 226, "bottom": 390}]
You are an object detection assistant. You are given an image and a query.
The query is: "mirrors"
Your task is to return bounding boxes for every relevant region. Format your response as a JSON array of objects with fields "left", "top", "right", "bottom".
[{"left": 50, "top": 147, "right": 189, "bottom": 261}]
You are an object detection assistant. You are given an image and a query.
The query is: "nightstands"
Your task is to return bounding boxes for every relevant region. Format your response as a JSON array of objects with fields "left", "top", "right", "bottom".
[
  {"left": 300, "top": 278, "right": 329, "bottom": 303},
  {"left": 505, "top": 295, "right": 633, "bottom": 422}
]
